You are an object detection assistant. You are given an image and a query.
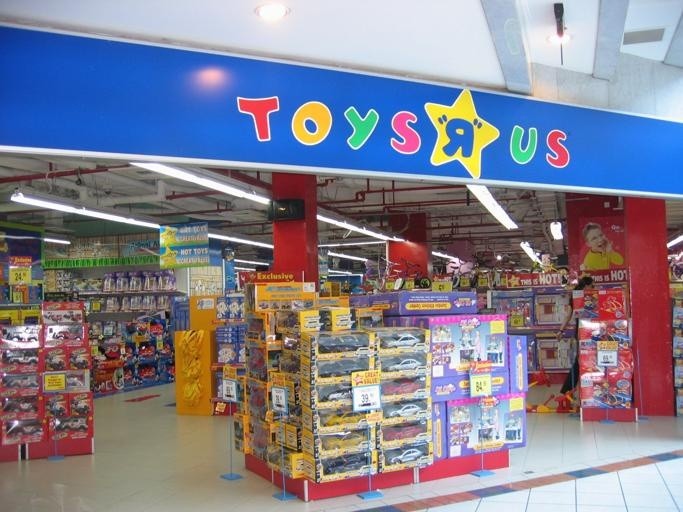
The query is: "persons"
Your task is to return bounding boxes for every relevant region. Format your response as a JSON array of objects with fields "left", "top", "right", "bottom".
[
  {"left": 581, "top": 222, "right": 624, "bottom": 270},
  {"left": 556, "top": 277, "right": 593, "bottom": 394}
]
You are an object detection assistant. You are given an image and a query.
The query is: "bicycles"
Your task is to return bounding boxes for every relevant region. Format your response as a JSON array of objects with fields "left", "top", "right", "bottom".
[{"left": 373, "top": 250, "right": 568, "bottom": 292}]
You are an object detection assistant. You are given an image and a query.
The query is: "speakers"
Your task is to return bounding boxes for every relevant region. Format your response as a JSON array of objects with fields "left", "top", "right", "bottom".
[{"left": 267, "top": 199, "right": 304, "bottom": 221}]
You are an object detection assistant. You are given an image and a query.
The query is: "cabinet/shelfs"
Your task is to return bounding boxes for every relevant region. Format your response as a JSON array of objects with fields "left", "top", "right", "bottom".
[{"left": 42, "top": 265, "right": 188, "bottom": 337}]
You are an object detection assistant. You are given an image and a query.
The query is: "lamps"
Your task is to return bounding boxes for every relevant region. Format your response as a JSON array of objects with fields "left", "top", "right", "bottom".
[
  {"left": 315, "top": 205, "right": 467, "bottom": 277},
  {"left": 11, "top": 187, "right": 129, "bottom": 249},
  {"left": 466, "top": 183, "right": 563, "bottom": 276},
  {"left": 130, "top": 162, "right": 272, "bottom": 274}
]
